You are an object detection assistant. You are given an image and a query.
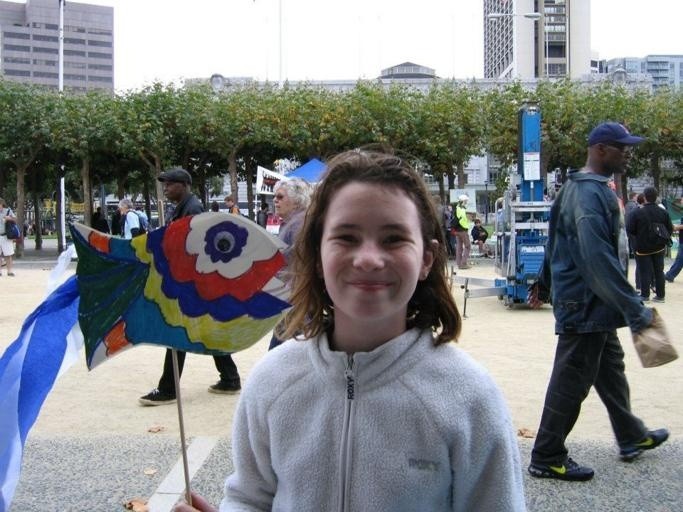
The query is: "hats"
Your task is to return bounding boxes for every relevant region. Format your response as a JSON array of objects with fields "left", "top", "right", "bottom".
[
  {"left": 460, "top": 195, "right": 469, "bottom": 201},
  {"left": 586, "top": 122, "right": 644, "bottom": 146},
  {"left": 158, "top": 169, "right": 192, "bottom": 185}
]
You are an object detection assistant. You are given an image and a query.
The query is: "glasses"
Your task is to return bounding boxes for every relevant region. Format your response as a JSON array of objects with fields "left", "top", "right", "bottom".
[{"left": 272, "top": 195, "right": 283, "bottom": 200}]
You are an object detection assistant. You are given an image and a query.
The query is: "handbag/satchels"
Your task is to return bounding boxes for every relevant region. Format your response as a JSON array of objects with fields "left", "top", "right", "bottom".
[
  {"left": 649, "top": 222, "right": 670, "bottom": 245},
  {"left": 449, "top": 216, "right": 459, "bottom": 226},
  {"left": 5, "top": 220, "right": 21, "bottom": 239}
]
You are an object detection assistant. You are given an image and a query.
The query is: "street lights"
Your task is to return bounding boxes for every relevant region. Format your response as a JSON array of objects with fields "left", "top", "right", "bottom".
[{"left": 485, "top": 179, "right": 491, "bottom": 225}]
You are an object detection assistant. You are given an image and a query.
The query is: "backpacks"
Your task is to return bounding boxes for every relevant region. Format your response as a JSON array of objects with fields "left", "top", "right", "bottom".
[{"left": 128, "top": 210, "right": 151, "bottom": 233}]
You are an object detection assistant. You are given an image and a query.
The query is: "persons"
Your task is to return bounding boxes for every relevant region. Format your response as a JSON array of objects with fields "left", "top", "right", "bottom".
[
  {"left": 625, "top": 186, "right": 683, "bottom": 302},
  {"left": 92, "top": 198, "right": 150, "bottom": 240},
  {"left": 267, "top": 177, "right": 327, "bottom": 349},
  {"left": 170, "top": 146, "right": 528, "bottom": 512},
  {"left": 258, "top": 203, "right": 269, "bottom": 228},
  {"left": 527, "top": 121, "right": 670, "bottom": 484},
  {"left": 0, "top": 198, "right": 17, "bottom": 276},
  {"left": 212, "top": 201, "right": 220, "bottom": 214},
  {"left": 429, "top": 194, "right": 503, "bottom": 269},
  {"left": 226, "top": 195, "right": 240, "bottom": 214},
  {"left": 139, "top": 168, "right": 243, "bottom": 404}
]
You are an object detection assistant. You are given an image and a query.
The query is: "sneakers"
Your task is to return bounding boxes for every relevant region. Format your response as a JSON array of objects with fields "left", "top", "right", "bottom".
[
  {"left": 619, "top": 428, "right": 669, "bottom": 459},
  {"left": 8, "top": 272, "right": 14, "bottom": 276},
  {"left": 653, "top": 296, "right": 665, "bottom": 303},
  {"left": 208, "top": 380, "right": 241, "bottom": 393},
  {"left": 639, "top": 295, "right": 649, "bottom": 303},
  {"left": 457, "top": 264, "right": 470, "bottom": 269},
  {"left": 138, "top": 387, "right": 178, "bottom": 406},
  {"left": 529, "top": 458, "right": 596, "bottom": 480}
]
